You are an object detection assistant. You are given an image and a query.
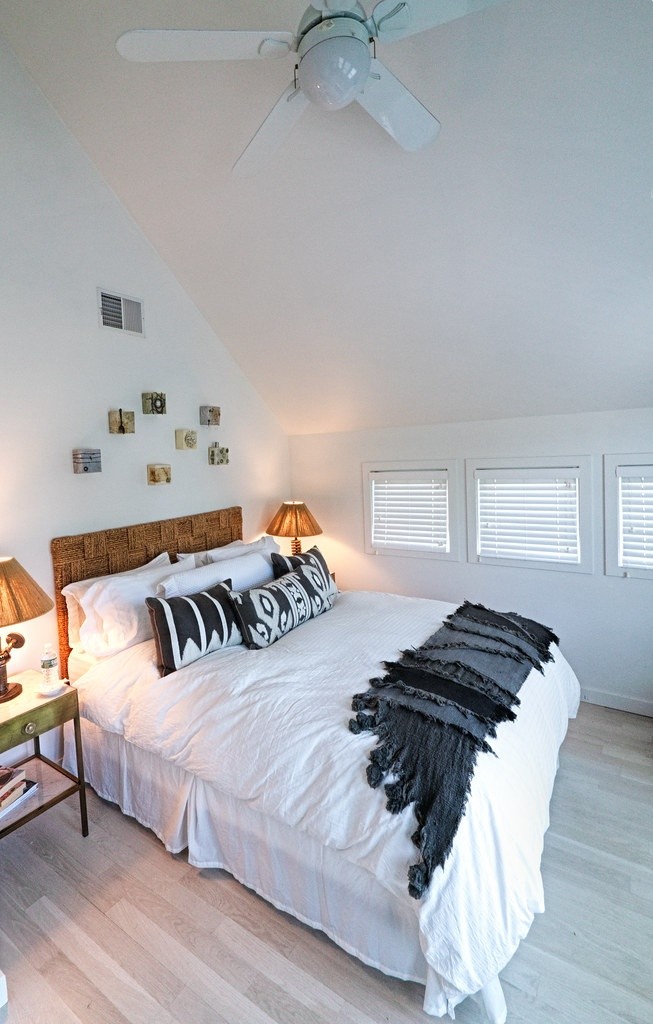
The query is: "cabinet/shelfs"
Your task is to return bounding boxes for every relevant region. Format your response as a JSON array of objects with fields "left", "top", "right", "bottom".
[{"left": 0, "top": 670, "right": 89, "bottom": 839}]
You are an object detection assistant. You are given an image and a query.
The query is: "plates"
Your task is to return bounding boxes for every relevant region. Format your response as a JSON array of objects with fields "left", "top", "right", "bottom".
[{"left": 37, "top": 678, "right": 70, "bottom": 695}]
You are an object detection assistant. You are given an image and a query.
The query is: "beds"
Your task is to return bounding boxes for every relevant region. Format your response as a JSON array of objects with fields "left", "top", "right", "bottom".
[{"left": 51, "top": 506, "right": 580, "bottom": 1024}]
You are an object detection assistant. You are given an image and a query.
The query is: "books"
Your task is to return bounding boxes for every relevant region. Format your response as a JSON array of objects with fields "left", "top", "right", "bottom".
[{"left": 0, "top": 764, "right": 39, "bottom": 819}]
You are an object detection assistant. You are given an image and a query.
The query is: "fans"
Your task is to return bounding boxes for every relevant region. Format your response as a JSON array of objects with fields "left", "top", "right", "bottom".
[{"left": 115, "top": 0, "right": 510, "bottom": 172}]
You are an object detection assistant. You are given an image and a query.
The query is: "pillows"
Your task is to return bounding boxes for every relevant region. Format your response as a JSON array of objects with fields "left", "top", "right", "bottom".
[
  {"left": 146, "top": 578, "right": 243, "bottom": 678},
  {"left": 227, "top": 557, "right": 334, "bottom": 650},
  {"left": 200, "top": 536, "right": 280, "bottom": 565},
  {"left": 79, "top": 554, "right": 197, "bottom": 656},
  {"left": 176, "top": 540, "right": 243, "bottom": 568},
  {"left": 156, "top": 549, "right": 275, "bottom": 599},
  {"left": 271, "top": 545, "right": 340, "bottom": 596},
  {"left": 61, "top": 552, "right": 171, "bottom": 654}
]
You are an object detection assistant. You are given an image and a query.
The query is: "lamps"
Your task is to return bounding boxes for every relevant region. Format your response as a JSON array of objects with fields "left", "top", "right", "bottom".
[
  {"left": 265, "top": 501, "right": 323, "bottom": 555},
  {"left": 0, "top": 556, "right": 55, "bottom": 703},
  {"left": 297, "top": 17, "right": 373, "bottom": 112}
]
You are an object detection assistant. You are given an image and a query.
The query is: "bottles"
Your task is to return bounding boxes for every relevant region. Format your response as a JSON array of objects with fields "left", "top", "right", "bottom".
[{"left": 41, "top": 643, "right": 59, "bottom": 691}]
